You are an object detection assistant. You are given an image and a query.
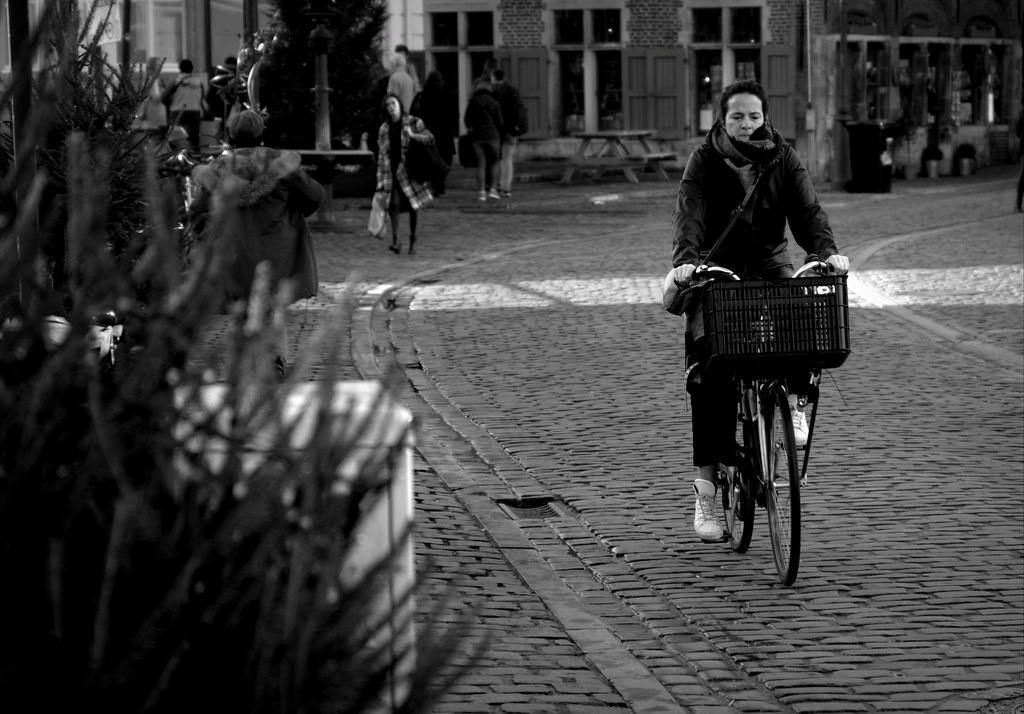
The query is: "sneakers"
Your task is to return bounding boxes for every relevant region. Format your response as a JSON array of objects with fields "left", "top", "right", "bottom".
[
  {"left": 691, "top": 478, "right": 724, "bottom": 539},
  {"left": 785, "top": 394, "right": 809, "bottom": 449}
]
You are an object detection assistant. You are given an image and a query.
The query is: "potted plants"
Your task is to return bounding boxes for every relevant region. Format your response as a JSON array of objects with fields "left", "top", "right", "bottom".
[
  {"left": 957, "top": 143, "right": 976, "bottom": 177},
  {"left": 922, "top": 146, "right": 943, "bottom": 178}
]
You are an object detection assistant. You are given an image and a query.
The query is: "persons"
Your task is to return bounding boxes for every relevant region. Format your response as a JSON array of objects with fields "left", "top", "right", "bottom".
[
  {"left": 162, "top": 60, "right": 205, "bottom": 149},
  {"left": 189, "top": 110, "right": 326, "bottom": 383},
  {"left": 207, "top": 58, "right": 248, "bottom": 122},
  {"left": 1015, "top": 98, "right": 1024, "bottom": 213},
  {"left": 386, "top": 44, "right": 459, "bottom": 198},
  {"left": 464, "top": 57, "right": 519, "bottom": 201},
  {"left": 672, "top": 79, "right": 849, "bottom": 540},
  {"left": 376, "top": 93, "right": 436, "bottom": 254},
  {"left": 137, "top": 58, "right": 168, "bottom": 131}
]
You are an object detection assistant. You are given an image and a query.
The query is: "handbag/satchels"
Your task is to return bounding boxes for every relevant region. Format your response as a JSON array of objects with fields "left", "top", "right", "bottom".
[
  {"left": 502, "top": 86, "right": 527, "bottom": 136},
  {"left": 459, "top": 135, "right": 479, "bottom": 167},
  {"left": 407, "top": 117, "right": 437, "bottom": 178},
  {"left": 369, "top": 192, "right": 389, "bottom": 240},
  {"left": 664, "top": 269, "right": 693, "bottom": 314}
]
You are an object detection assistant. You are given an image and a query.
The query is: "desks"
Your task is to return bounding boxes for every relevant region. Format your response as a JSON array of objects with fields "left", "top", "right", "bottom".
[{"left": 559, "top": 130, "right": 670, "bottom": 184}]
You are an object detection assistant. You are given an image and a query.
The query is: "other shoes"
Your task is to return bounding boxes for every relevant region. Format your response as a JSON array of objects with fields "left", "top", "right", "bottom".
[
  {"left": 478, "top": 193, "right": 486, "bottom": 200},
  {"left": 488, "top": 191, "right": 500, "bottom": 199},
  {"left": 389, "top": 245, "right": 401, "bottom": 253},
  {"left": 409, "top": 245, "right": 415, "bottom": 255}
]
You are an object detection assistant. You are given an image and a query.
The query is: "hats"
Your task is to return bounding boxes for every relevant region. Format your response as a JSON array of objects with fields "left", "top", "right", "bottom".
[{"left": 427, "top": 70, "right": 444, "bottom": 85}]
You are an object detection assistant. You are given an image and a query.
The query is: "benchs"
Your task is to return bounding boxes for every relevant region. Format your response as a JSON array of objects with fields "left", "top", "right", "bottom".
[
  {"left": 626, "top": 151, "right": 679, "bottom": 160},
  {"left": 563, "top": 152, "right": 613, "bottom": 163}
]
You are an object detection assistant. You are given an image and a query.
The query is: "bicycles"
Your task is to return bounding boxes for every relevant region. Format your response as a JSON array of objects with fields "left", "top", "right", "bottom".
[{"left": 674, "top": 252, "right": 850, "bottom": 587}]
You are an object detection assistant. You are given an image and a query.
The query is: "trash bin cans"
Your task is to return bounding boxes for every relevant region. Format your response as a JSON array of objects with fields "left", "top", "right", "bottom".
[{"left": 842, "top": 119, "right": 897, "bottom": 194}]
